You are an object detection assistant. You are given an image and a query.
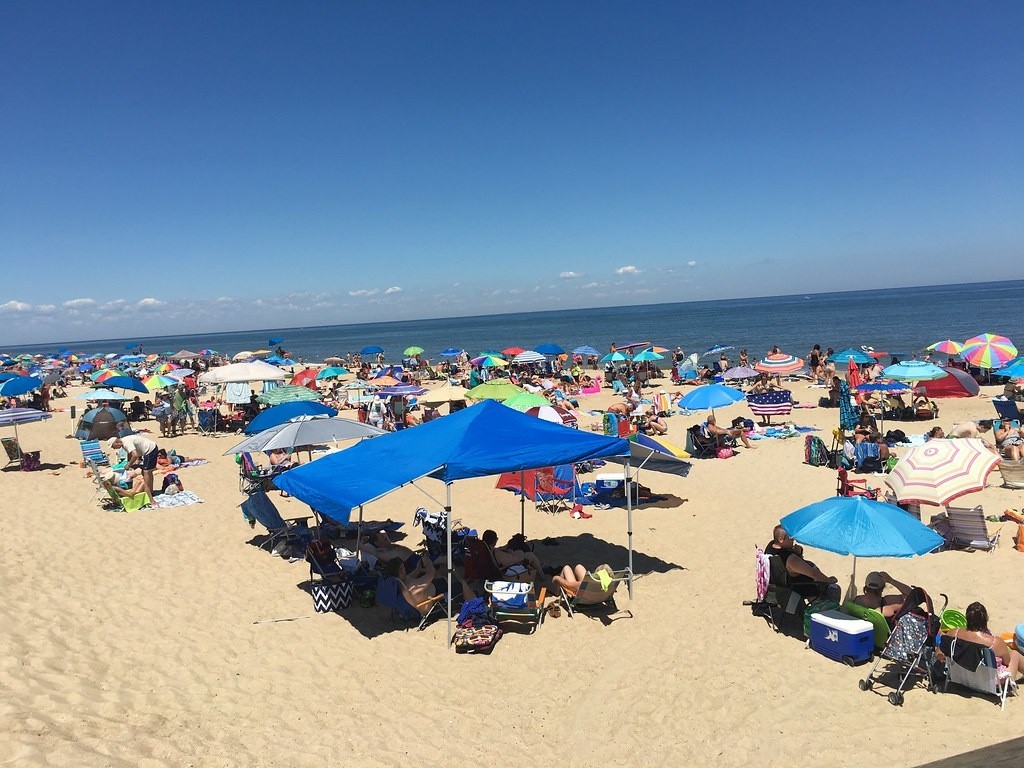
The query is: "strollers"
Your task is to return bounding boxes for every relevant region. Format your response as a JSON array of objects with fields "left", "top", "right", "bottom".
[{"left": 858, "top": 585, "right": 950, "bottom": 706}]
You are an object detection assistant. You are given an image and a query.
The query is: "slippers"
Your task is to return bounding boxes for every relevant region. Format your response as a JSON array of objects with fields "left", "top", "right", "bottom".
[
  {"left": 553, "top": 606, "right": 561, "bottom": 617},
  {"left": 547, "top": 602, "right": 555, "bottom": 617}
]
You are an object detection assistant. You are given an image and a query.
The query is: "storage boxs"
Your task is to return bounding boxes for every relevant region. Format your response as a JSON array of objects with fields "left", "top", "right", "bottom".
[
  {"left": 595, "top": 473, "right": 626, "bottom": 492},
  {"left": 810, "top": 609, "right": 874, "bottom": 666},
  {"left": 394, "top": 422, "right": 404, "bottom": 431}
]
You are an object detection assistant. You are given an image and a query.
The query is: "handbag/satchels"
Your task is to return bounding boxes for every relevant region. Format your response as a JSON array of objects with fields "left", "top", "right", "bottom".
[
  {"left": 715, "top": 445, "right": 732, "bottom": 459},
  {"left": 310, "top": 573, "right": 354, "bottom": 614},
  {"left": 152, "top": 407, "right": 165, "bottom": 416}
]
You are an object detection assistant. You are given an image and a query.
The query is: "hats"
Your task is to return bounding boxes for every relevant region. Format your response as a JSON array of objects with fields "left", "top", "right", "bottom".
[
  {"left": 865, "top": 572, "right": 885, "bottom": 589},
  {"left": 374, "top": 395, "right": 380, "bottom": 404},
  {"left": 107, "top": 437, "right": 117, "bottom": 449},
  {"left": 161, "top": 391, "right": 170, "bottom": 395},
  {"left": 1008, "top": 376, "right": 1019, "bottom": 384}
]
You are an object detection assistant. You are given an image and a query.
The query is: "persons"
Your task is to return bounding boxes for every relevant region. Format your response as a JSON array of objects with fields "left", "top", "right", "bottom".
[{"left": 0, "top": 344, "right": 1024, "bottom": 688}]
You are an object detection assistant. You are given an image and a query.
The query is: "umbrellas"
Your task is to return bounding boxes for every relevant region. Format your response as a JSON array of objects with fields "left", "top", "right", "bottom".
[{"left": 1, "top": 334, "right": 1023, "bottom": 598}]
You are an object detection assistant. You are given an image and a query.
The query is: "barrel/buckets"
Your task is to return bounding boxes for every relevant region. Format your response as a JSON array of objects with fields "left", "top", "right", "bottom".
[
  {"left": 940, "top": 610, "right": 967, "bottom": 634},
  {"left": 357, "top": 586, "right": 375, "bottom": 608}
]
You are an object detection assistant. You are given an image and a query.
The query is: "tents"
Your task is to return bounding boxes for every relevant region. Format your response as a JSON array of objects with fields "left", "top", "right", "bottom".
[{"left": 272, "top": 399, "right": 633, "bottom": 647}]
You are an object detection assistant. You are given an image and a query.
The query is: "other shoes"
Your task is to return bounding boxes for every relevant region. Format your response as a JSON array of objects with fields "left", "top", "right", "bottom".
[{"left": 595, "top": 502, "right": 610, "bottom": 509}]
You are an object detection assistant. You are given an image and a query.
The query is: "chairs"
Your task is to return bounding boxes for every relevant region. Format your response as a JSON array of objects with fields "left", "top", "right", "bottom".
[
  {"left": 0, "top": 437, "right": 42, "bottom": 468},
  {"left": 80, "top": 361, "right": 1024, "bottom": 710}
]
